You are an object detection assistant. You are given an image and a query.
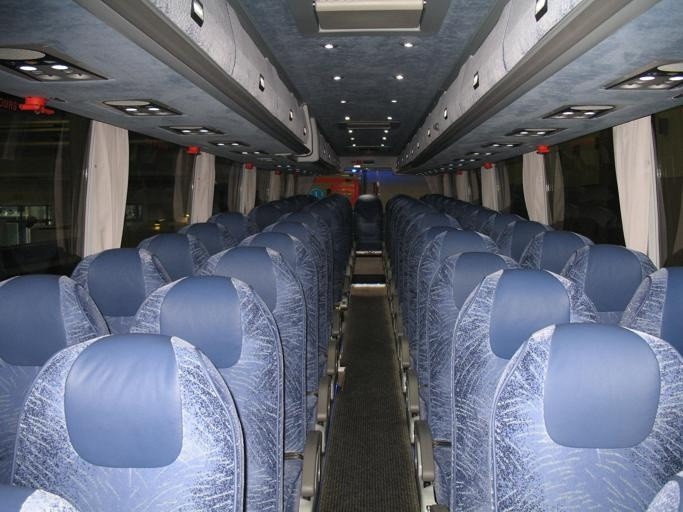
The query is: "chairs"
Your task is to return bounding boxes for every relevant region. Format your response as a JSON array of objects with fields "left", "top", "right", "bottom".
[
  {"left": 138, "top": 213, "right": 348, "bottom": 455},
  {"left": 249, "top": 194, "right": 355, "bottom": 356},
  {"left": 1, "top": 245, "right": 322, "bottom": 512},
  {"left": 380, "top": 192, "right": 682, "bottom": 512}
]
